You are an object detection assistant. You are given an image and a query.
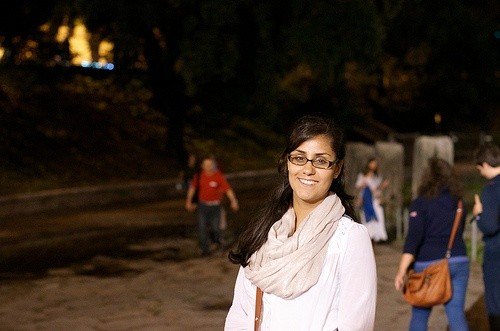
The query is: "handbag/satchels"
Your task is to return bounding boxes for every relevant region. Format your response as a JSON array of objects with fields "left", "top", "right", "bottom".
[
  {"left": 192, "top": 186, "right": 200, "bottom": 204},
  {"left": 400, "top": 258, "right": 452, "bottom": 308}
]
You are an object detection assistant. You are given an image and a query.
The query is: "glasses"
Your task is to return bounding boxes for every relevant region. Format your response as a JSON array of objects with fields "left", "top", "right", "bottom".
[{"left": 287, "top": 153, "right": 338, "bottom": 169}]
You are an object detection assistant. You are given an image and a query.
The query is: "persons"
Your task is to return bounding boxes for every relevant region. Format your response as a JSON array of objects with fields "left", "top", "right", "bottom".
[
  {"left": 181, "top": 151, "right": 239, "bottom": 257},
  {"left": 394, "top": 143, "right": 500, "bottom": 331},
  {"left": 223, "top": 115, "right": 376, "bottom": 331},
  {"left": 355, "top": 157, "right": 390, "bottom": 242}
]
